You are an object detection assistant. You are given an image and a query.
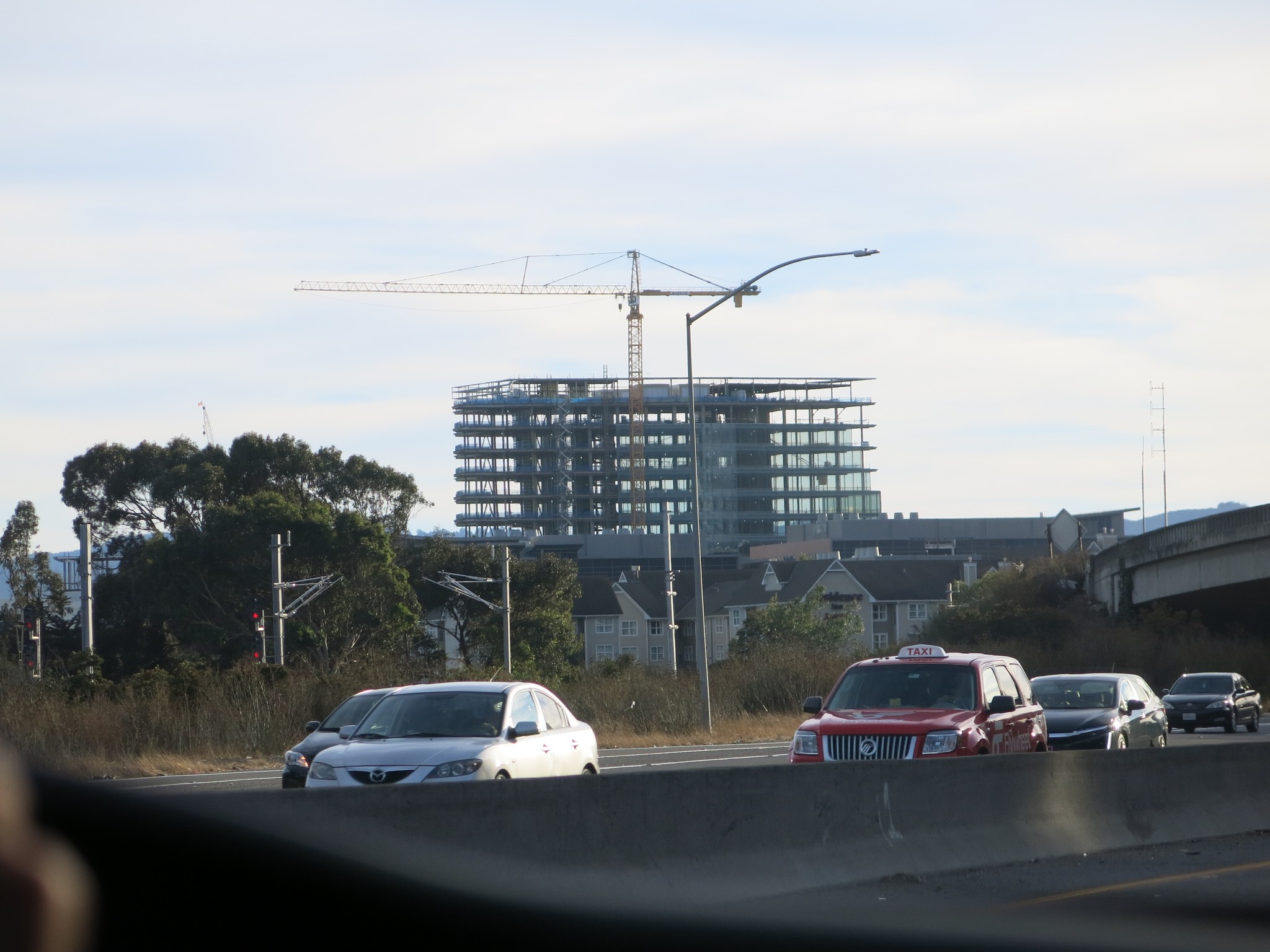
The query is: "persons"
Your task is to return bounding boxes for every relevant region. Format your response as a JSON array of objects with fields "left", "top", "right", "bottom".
[
  {"left": 937, "top": 675, "right": 972, "bottom": 707},
  {"left": 1094, "top": 692, "right": 1108, "bottom": 707}
]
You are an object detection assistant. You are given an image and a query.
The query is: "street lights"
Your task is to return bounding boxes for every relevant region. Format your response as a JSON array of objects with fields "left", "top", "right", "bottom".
[{"left": 684, "top": 246, "right": 883, "bottom": 735}]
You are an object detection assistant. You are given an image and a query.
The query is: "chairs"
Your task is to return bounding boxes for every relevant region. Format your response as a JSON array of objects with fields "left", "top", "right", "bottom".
[{"left": 1066, "top": 691, "right": 1080, "bottom": 698}]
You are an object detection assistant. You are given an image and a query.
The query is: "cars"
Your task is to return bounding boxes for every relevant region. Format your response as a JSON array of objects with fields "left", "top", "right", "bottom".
[
  {"left": 1030, "top": 672, "right": 1168, "bottom": 749},
  {"left": 304, "top": 681, "right": 601, "bottom": 788},
  {"left": 281, "top": 687, "right": 405, "bottom": 788},
  {"left": 1161, "top": 672, "right": 1261, "bottom": 733}
]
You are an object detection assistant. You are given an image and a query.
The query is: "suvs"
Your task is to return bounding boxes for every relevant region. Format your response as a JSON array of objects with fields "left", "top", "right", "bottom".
[{"left": 789, "top": 643, "right": 1048, "bottom": 763}]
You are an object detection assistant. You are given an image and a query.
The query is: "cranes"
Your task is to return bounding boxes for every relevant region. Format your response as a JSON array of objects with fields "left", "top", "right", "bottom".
[
  {"left": 202, "top": 405, "right": 215, "bottom": 447},
  {"left": 289, "top": 249, "right": 761, "bottom": 535}
]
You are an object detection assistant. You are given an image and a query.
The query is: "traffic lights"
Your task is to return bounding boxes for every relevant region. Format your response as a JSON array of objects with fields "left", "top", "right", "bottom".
[
  {"left": 24, "top": 644, "right": 33, "bottom": 671},
  {"left": 249, "top": 634, "right": 264, "bottom": 661},
  {"left": 249, "top": 595, "right": 264, "bottom": 622},
  {"left": 23, "top": 604, "right": 37, "bottom": 632}
]
